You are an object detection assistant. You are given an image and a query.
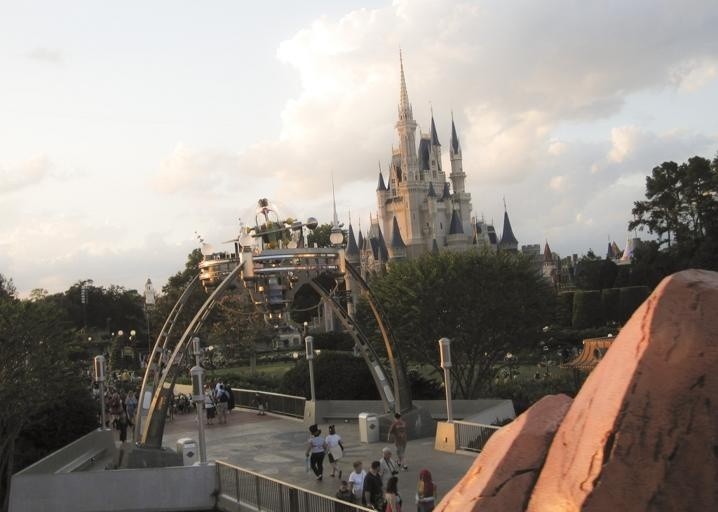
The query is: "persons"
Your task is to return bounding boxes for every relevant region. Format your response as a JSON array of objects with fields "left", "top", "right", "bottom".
[
  {"left": 334, "top": 480, "right": 356, "bottom": 511},
  {"left": 382, "top": 474, "right": 402, "bottom": 512},
  {"left": 362, "top": 459, "right": 386, "bottom": 511},
  {"left": 252, "top": 391, "right": 267, "bottom": 417},
  {"left": 306, "top": 423, "right": 329, "bottom": 482},
  {"left": 98, "top": 383, "right": 139, "bottom": 445},
  {"left": 347, "top": 459, "right": 367, "bottom": 507},
  {"left": 325, "top": 424, "right": 344, "bottom": 479},
  {"left": 415, "top": 468, "right": 438, "bottom": 511},
  {"left": 379, "top": 446, "right": 400, "bottom": 494},
  {"left": 205, "top": 376, "right": 236, "bottom": 427},
  {"left": 388, "top": 412, "right": 409, "bottom": 472}
]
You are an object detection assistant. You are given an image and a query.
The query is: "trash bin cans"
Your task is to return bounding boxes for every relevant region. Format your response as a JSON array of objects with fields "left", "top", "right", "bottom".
[
  {"left": 176, "top": 438, "right": 199, "bottom": 466},
  {"left": 358, "top": 412, "right": 379, "bottom": 443}
]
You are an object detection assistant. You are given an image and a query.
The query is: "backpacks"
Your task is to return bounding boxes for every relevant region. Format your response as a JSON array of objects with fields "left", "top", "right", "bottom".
[{"left": 218, "top": 390, "right": 228, "bottom": 402}]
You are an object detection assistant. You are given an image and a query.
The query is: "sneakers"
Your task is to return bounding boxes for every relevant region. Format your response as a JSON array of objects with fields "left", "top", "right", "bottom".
[
  {"left": 395, "top": 460, "right": 408, "bottom": 471},
  {"left": 315, "top": 469, "right": 343, "bottom": 481}
]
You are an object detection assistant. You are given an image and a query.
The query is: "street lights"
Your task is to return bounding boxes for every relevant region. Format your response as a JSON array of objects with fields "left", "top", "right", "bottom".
[
  {"left": 143, "top": 279, "right": 156, "bottom": 358},
  {"left": 507, "top": 352, "right": 512, "bottom": 379},
  {"left": 95, "top": 355, "right": 106, "bottom": 430},
  {"left": 190, "top": 337, "right": 207, "bottom": 465},
  {"left": 438, "top": 338, "right": 453, "bottom": 422},
  {"left": 305, "top": 336, "right": 316, "bottom": 402}
]
